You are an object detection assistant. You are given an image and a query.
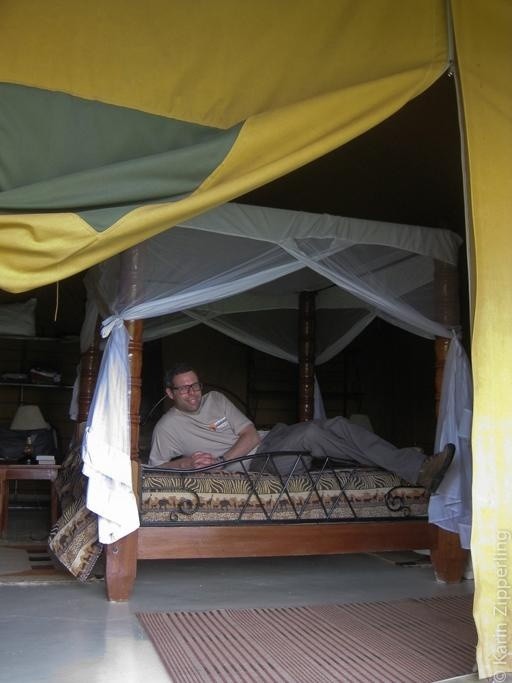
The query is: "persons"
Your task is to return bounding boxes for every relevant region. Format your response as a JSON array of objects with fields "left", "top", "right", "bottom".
[{"left": 148, "top": 363, "right": 456, "bottom": 493}]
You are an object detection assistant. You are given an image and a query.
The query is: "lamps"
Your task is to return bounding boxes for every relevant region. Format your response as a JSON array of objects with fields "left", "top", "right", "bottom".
[{"left": 8, "top": 402, "right": 51, "bottom": 463}]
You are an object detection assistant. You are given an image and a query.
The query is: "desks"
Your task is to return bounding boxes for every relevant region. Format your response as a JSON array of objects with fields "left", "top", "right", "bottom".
[{"left": 0, "top": 460, "right": 62, "bottom": 539}]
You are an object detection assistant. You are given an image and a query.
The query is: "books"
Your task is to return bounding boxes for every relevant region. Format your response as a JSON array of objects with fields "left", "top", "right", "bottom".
[
  {"left": 39, "top": 461, "right": 55, "bottom": 465},
  {"left": 36, "top": 455, "right": 56, "bottom": 460}
]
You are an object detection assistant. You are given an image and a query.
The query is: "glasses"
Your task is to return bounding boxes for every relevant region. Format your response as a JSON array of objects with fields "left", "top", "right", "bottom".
[{"left": 172, "top": 382, "right": 203, "bottom": 394}]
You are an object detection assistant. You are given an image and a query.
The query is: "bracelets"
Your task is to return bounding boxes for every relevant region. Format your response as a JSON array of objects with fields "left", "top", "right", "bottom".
[{"left": 219, "top": 457, "right": 226, "bottom": 469}]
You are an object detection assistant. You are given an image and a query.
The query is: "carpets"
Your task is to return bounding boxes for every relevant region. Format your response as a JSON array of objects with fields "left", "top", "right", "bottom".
[
  {"left": 133, "top": 593, "right": 482, "bottom": 682},
  {"left": 1, "top": 539, "right": 107, "bottom": 587}
]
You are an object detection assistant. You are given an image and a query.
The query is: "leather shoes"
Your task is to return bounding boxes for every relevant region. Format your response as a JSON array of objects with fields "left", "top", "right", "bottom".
[{"left": 416, "top": 442, "right": 456, "bottom": 494}]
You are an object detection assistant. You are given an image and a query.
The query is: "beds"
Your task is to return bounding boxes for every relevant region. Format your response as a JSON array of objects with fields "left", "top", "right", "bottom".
[{"left": 52, "top": 198, "right": 470, "bottom": 601}]
